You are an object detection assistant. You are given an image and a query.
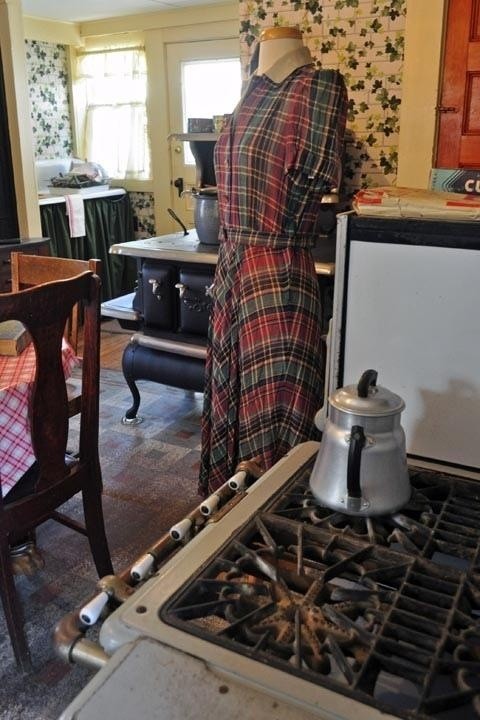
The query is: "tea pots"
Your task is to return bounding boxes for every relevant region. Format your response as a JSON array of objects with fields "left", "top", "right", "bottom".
[{"left": 309, "top": 370, "right": 415, "bottom": 518}]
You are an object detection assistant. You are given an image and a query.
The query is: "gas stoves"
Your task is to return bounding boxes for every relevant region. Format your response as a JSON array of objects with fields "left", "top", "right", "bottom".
[{"left": 76, "top": 438, "right": 480, "bottom": 720}]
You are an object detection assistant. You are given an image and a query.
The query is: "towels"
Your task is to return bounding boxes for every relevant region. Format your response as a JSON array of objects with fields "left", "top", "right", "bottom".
[{"left": 66, "top": 194, "right": 87, "bottom": 238}]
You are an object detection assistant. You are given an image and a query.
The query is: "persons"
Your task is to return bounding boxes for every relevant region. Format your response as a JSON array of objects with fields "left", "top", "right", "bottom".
[{"left": 193, "top": 26, "right": 348, "bottom": 507}]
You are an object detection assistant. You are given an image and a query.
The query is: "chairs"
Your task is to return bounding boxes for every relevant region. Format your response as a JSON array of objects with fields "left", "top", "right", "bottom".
[
  {"left": 0, "top": 270, "right": 114, "bottom": 678},
  {"left": 10, "top": 252, "right": 102, "bottom": 357}
]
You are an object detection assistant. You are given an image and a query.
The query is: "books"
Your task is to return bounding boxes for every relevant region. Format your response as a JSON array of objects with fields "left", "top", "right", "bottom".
[{"left": 0, "top": 320, "right": 33, "bottom": 357}]
